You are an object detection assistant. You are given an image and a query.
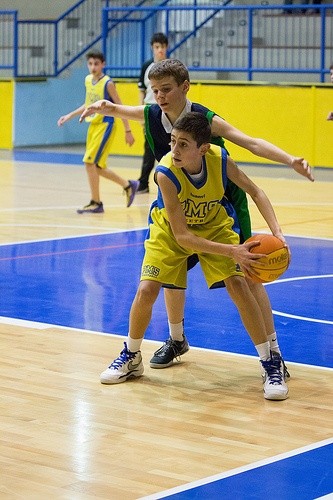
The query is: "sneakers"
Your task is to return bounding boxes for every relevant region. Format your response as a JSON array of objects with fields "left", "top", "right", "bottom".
[
  {"left": 263, "top": 350, "right": 289, "bottom": 399},
  {"left": 77, "top": 200, "right": 103, "bottom": 213},
  {"left": 135, "top": 182, "right": 149, "bottom": 193},
  {"left": 149, "top": 335, "right": 189, "bottom": 369},
  {"left": 124, "top": 180, "right": 139, "bottom": 207},
  {"left": 261, "top": 351, "right": 290, "bottom": 384},
  {"left": 100, "top": 342, "right": 144, "bottom": 383}
]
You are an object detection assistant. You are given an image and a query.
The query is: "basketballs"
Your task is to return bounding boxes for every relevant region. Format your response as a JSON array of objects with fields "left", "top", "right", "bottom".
[{"left": 243, "top": 234, "right": 289, "bottom": 283}]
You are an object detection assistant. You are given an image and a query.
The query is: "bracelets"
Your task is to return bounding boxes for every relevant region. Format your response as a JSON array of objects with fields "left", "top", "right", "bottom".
[{"left": 126, "top": 130, "right": 131, "bottom": 133}]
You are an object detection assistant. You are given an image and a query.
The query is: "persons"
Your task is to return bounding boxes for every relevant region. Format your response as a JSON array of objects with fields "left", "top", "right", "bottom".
[
  {"left": 99, "top": 112, "right": 289, "bottom": 401},
  {"left": 137, "top": 32, "right": 169, "bottom": 193},
  {"left": 58, "top": 49, "right": 140, "bottom": 214},
  {"left": 79, "top": 58, "right": 315, "bottom": 384}
]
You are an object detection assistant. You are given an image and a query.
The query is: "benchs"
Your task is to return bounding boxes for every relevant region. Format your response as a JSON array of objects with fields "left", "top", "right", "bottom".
[{"left": 0, "top": 13, "right": 332, "bottom": 81}]
